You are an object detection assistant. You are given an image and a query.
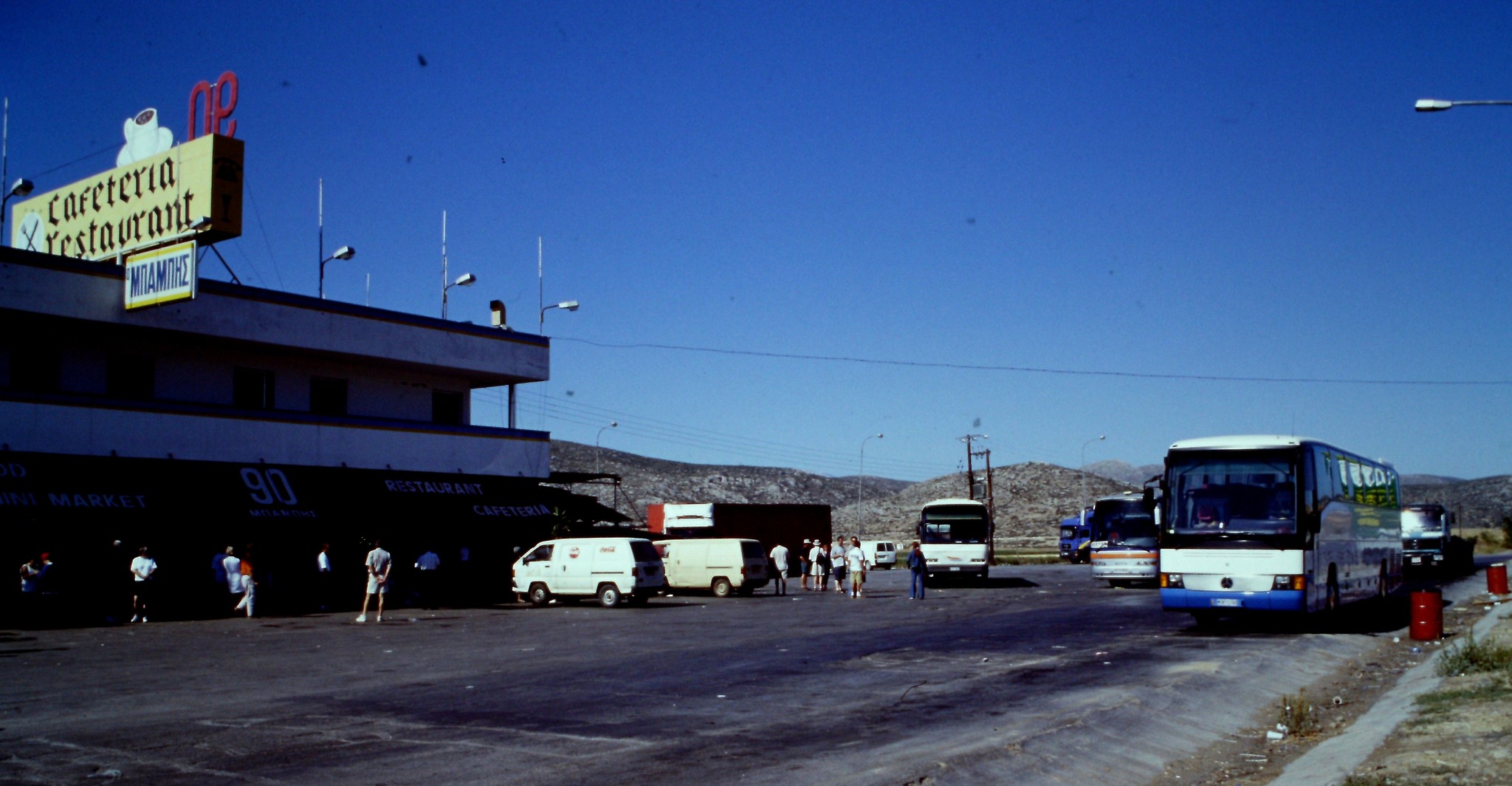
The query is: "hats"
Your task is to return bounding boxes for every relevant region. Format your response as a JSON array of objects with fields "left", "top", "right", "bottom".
[
  {"left": 804, "top": 539, "right": 812, "bottom": 543},
  {"left": 41, "top": 552, "right": 50, "bottom": 560},
  {"left": 813, "top": 540, "right": 821, "bottom": 546}
]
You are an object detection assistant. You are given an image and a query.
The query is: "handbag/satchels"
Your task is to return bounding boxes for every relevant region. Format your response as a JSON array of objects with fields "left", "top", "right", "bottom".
[{"left": 816, "top": 547, "right": 825, "bottom": 565}]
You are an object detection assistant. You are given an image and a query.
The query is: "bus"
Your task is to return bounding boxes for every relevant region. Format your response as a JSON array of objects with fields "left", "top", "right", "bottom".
[
  {"left": 1081, "top": 491, "right": 1156, "bottom": 589},
  {"left": 916, "top": 497, "right": 996, "bottom": 589},
  {"left": 1400, "top": 502, "right": 1453, "bottom": 574},
  {"left": 1141, "top": 431, "right": 1404, "bottom": 621}
]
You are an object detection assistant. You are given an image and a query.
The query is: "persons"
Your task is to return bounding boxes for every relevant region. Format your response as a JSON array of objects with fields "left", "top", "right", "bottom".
[
  {"left": 808, "top": 540, "right": 826, "bottom": 591},
  {"left": 817, "top": 543, "right": 831, "bottom": 591},
  {"left": 513, "top": 548, "right": 528, "bottom": 603},
  {"left": 414, "top": 546, "right": 441, "bottom": 610},
  {"left": 20, "top": 555, "right": 43, "bottom": 630},
  {"left": 847, "top": 540, "right": 866, "bottom": 599},
  {"left": 799, "top": 539, "right": 812, "bottom": 591},
  {"left": 32, "top": 551, "right": 58, "bottom": 630},
  {"left": 222, "top": 546, "right": 247, "bottom": 617},
  {"left": 318, "top": 544, "right": 334, "bottom": 614},
  {"left": 210, "top": 549, "right": 235, "bottom": 620},
  {"left": 844, "top": 536, "right": 862, "bottom": 595},
  {"left": 830, "top": 535, "right": 846, "bottom": 595},
  {"left": 357, "top": 539, "right": 391, "bottom": 623},
  {"left": 129, "top": 545, "right": 157, "bottom": 623},
  {"left": 906, "top": 541, "right": 927, "bottom": 600},
  {"left": 233, "top": 547, "right": 258, "bottom": 618},
  {"left": 769, "top": 539, "right": 791, "bottom": 597}
]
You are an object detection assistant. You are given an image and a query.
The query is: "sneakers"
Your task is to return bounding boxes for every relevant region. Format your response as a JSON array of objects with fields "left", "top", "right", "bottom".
[
  {"left": 356, "top": 616, "right": 367, "bottom": 622},
  {"left": 131, "top": 614, "right": 138, "bottom": 622},
  {"left": 142, "top": 617, "right": 148, "bottom": 623},
  {"left": 377, "top": 617, "right": 382, "bottom": 621}
]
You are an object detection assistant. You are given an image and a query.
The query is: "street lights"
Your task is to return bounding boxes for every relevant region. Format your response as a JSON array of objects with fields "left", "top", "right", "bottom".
[
  {"left": 595, "top": 422, "right": 618, "bottom": 526},
  {"left": 1081, "top": 435, "right": 1105, "bottom": 509},
  {"left": 857, "top": 433, "right": 885, "bottom": 541}
]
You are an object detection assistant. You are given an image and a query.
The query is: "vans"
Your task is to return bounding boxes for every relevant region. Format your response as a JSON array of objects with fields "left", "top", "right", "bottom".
[
  {"left": 859, "top": 540, "right": 897, "bottom": 571},
  {"left": 509, "top": 537, "right": 666, "bottom": 608},
  {"left": 652, "top": 539, "right": 768, "bottom": 598}
]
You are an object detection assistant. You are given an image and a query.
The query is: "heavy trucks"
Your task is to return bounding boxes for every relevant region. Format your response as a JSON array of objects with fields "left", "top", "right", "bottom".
[{"left": 1059, "top": 508, "right": 1091, "bottom": 565}]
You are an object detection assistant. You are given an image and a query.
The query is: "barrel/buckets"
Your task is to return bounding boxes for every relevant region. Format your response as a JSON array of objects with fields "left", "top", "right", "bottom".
[
  {"left": 1485, "top": 562, "right": 1508, "bottom": 594},
  {"left": 1408, "top": 586, "right": 1443, "bottom": 640}
]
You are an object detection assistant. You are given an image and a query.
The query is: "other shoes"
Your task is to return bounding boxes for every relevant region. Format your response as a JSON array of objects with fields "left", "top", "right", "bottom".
[
  {"left": 773, "top": 587, "right": 924, "bottom": 600},
  {"left": 234, "top": 607, "right": 254, "bottom": 619}
]
